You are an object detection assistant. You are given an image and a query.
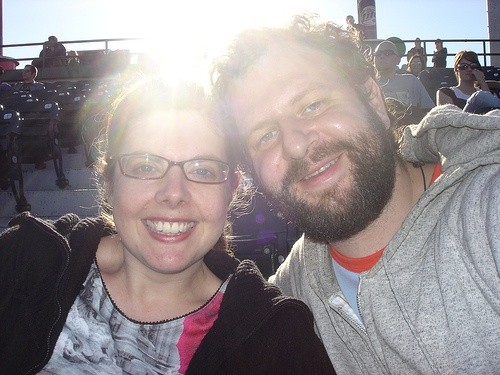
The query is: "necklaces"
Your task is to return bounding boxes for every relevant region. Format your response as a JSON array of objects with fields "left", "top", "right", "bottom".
[{"left": 406, "top": 159, "right": 427, "bottom": 191}]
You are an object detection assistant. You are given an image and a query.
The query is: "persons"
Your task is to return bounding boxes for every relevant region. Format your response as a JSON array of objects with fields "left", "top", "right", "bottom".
[
  {"left": 0, "top": 65, "right": 45, "bottom": 92},
  {"left": 210, "top": 13, "right": 500, "bottom": 375},
  {"left": 436, "top": 50, "right": 500, "bottom": 116},
  {"left": 373, "top": 41, "right": 436, "bottom": 128},
  {"left": 31, "top": 36, "right": 66, "bottom": 67},
  {"left": 346, "top": 16, "right": 366, "bottom": 56},
  {"left": 0, "top": 73, "right": 336, "bottom": 375},
  {"left": 405, "top": 54, "right": 429, "bottom": 94},
  {"left": 431, "top": 38, "right": 447, "bottom": 68},
  {"left": 407, "top": 37, "right": 427, "bottom": 68}
]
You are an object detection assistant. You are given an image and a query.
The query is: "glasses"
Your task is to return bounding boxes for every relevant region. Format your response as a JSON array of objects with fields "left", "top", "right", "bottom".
[
  {"left": 118, "top": 153, "right": 235, "bottom": 184},
  {"left": 374, "top": 50, "right": 397, "bottom": 56},
  {"left": 458, "top": 63, "right": 481, "bottom": 70}
]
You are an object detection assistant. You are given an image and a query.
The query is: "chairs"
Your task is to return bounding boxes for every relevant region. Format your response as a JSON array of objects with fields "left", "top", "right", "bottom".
[
  {"left": 0, "top": 79, "right": 121, "bottom": 212},
  {"left": 396, "top": 66, "right": 500, "bottom": 110},
  {"left": 226, "top": 190, "right": 307, "bottom": 276}
]
